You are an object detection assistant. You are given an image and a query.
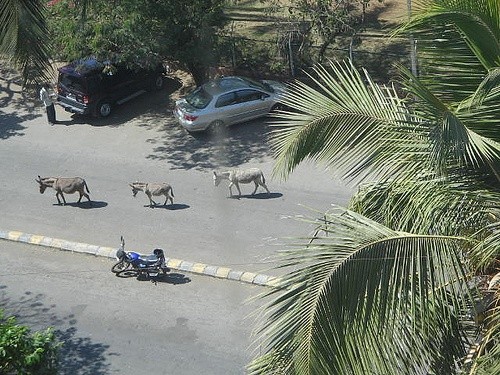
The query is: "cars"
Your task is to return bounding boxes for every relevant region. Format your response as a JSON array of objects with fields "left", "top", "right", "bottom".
[{"left": 174, "top": 76, "right": 288, "bottom": 137}]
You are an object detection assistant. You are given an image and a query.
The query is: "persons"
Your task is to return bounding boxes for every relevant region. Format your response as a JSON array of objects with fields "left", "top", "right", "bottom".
[{"left": 40, "top": 82, "right": 57, "bottom": 126}]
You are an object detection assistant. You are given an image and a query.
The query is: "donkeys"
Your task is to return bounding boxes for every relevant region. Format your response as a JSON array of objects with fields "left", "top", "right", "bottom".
[
  {"left": 35, "top": 175, "right": 92, "bottom": 206},
  {"left": 129, "top": 182, "right": 176, "bottom": 208},
  {"left": 212, "top": 169, "right": 271, "bottom": 199}
]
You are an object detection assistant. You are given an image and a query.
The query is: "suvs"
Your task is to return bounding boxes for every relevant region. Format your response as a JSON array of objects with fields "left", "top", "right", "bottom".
[{"left": 57, "top": 52, "right": 167, "bottom": 119}]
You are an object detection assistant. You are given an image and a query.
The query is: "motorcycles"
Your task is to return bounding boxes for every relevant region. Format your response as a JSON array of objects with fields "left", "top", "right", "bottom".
[{"left": 110, "top": 235, "right": 170, "bottom": 278}]
